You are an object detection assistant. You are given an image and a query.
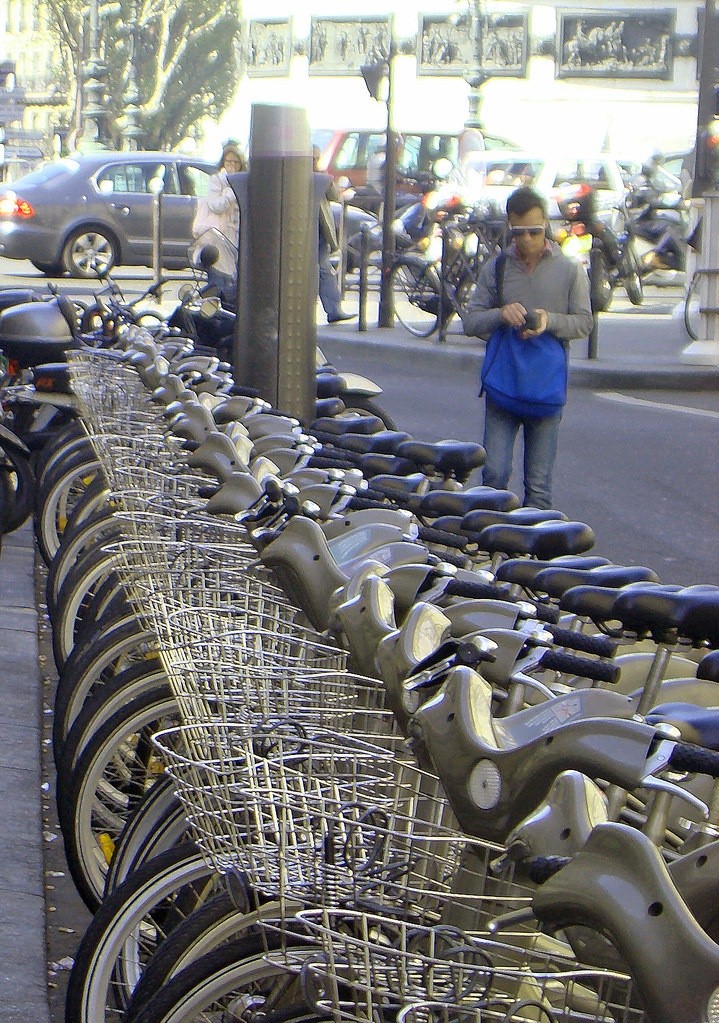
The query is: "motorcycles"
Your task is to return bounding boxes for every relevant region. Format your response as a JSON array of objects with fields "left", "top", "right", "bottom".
[{"left": 336, "top": 161, "right": 691, "bottom": 309}]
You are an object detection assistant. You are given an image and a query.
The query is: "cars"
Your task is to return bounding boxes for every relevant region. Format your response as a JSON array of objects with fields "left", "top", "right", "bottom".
[
  {"left": 316, "top": 124, "right": 533, "bottom": 187},
  {"left": 461, "top": 147, "right": 682, "bottom": 235},
  {"left": 0, "top": 150, "right": 381, "bottom": 280}
]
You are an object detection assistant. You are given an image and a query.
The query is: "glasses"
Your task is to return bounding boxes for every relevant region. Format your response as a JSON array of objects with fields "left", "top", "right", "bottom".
[
  {"left": 225, "top": 160, "right": 239, "bottom": 165},
  {"left": 508, "top": 220, "right": 546, "bottom": 235}
]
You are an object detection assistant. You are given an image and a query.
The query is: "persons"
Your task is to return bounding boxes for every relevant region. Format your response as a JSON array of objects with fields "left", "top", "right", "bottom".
[
  {"left": 626, "top": 159, "right": 674, "bottom": 270},
  {"left": 567, "top": 193, "right": 618, "bottom": 359},
  {"left": 312, "top": 144, "right": 358, "bottom": 323},
  {"left": 464, "top": 188, "right": 593, "bottom": 511},
  {"left": 367, "top": 128, "right": 430, "bottom": 207},
  {"left": 192, "top": 144, "right": 246, "bottom": 288}
]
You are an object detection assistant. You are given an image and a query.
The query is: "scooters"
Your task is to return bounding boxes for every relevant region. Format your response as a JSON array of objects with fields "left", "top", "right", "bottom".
[{"left": 0, "top": 226, "right": 398, "bottom": 537}]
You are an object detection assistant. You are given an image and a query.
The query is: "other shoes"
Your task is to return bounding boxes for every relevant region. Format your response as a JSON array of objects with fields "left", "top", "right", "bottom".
[{"left": 327, "top": 310, "right": 358, "bottom": 324}]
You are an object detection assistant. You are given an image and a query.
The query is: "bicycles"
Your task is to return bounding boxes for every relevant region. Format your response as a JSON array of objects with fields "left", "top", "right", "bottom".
[
  {"left": 683, "top": 212, "right": 703, "bottom": 341},
  {"left": 384, "top": 220, "right": 491, "bottom": 337},
  {"left": 31, "top": 326, "right": 719, "bottom": 1023}
]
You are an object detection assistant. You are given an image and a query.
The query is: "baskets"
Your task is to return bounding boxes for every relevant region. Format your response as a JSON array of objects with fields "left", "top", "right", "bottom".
[{"left": 63, "top": 346, "right": 644, "bottom": 1023}]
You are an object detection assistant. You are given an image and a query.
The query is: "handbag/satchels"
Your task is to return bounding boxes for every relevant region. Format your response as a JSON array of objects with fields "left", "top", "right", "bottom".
[
  {"left": 192, "top": 174, "right": 227, "bottom": 239},
  {"left": 481, "top": 319, "right": 567, "bottom": 418}
]
